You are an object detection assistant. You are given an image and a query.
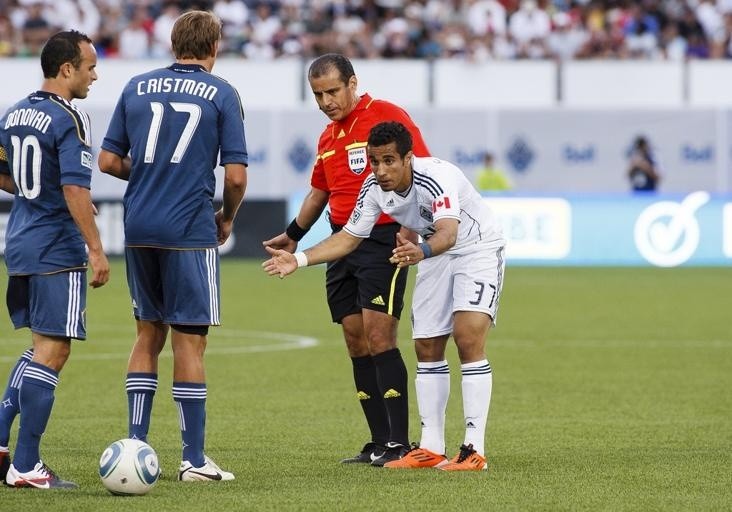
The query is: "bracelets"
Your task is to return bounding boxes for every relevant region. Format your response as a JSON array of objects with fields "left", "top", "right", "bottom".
[
  {"left": 293, "top": 251, "right": 308, "bottom": 268},
  {"left": 286, "top": 217, "right": 311, "bottom": 242},
  {"left": 418, "top": 242, "right": 433, "bottom": 259}
]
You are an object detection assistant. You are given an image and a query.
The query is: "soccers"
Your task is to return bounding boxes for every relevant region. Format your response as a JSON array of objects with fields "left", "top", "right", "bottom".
[{"left": 100, "top": 438, "right": 160, "bottom": 496}]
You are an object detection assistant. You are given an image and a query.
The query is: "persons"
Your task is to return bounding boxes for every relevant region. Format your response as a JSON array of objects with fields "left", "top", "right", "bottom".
[
  {"left": 261, "top": 120, "right": 509, "bottom": 473},
  {"left": 97, "top": 10, "right": 249, "bottom": 482},
  {"left": 1, "top": 30, "right": 111, "bottom": 491},
  {"left": 474, "top": 153, "right": 512, "bottom": 190},
  {"left": 625, "top": 137, "right": 662, "bottom": 192},
  {"left": 262, "top": 51, "right": 432, "bottom": 466}
]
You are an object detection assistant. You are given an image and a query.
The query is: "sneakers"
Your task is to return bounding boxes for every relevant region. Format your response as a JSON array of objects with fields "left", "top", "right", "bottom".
[
  {"left": 439, "top": 446, "right": 487, "bottom": 471},
  {"left": 177, "top": 454, "right": 235, "bottom": 481},
  {"left": 0, "top": 444, "right": 79, "bottom": 489},
  {"left": 341, "top": 441, "right": 448, "bottom": 468}
]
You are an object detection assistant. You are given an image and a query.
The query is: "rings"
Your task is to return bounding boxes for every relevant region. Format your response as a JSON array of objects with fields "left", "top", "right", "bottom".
[{"left": 406, "top": 256, "right": 409, "bottom": 261}]
